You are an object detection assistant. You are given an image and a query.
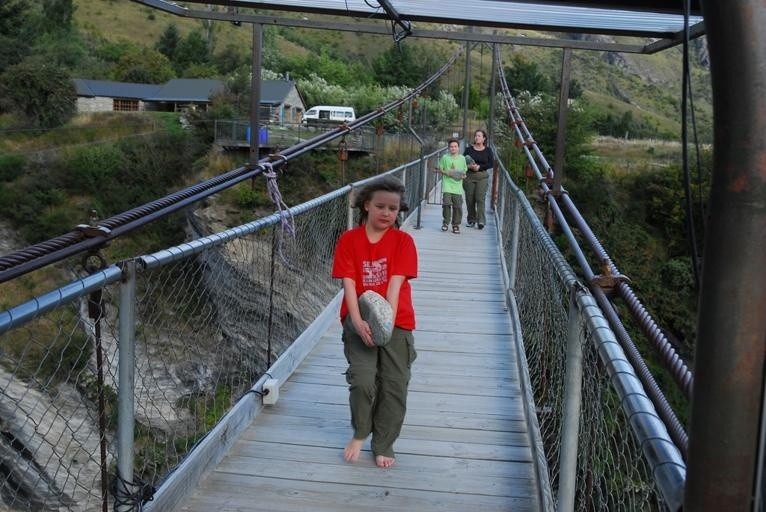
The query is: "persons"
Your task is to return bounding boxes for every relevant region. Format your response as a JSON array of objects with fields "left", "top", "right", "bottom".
[
  {"left": 328, "top": 172, "right": 417, "bottom": 469},
  {"left": 436, "top": 138, "right": 468, "bottom": 235},
  {"left": 461, "top": 129, "right": 494, "bottom": 229}
]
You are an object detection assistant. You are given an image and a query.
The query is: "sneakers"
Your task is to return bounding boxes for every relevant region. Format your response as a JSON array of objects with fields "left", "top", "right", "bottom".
[{"left": 477, "top": 222, "right": 485, "bottom": 230}]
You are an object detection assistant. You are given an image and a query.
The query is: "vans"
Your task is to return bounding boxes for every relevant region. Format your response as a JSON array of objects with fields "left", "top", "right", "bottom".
[{"left": 300, "top": 106, "right": 355, "bottom": 123}]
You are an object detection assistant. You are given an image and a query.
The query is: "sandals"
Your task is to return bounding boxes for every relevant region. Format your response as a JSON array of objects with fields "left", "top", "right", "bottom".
[
  {"left": 452, "top": 225, "right": 461, "bottom": 235},
  {"left": 441, "top": 222, "right": 449, "bottom": 232}
]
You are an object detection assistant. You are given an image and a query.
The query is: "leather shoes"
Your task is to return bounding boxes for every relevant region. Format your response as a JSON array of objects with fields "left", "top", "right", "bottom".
[{"left": 465, "top": 221, "right": 476, "bottom": 228}]
